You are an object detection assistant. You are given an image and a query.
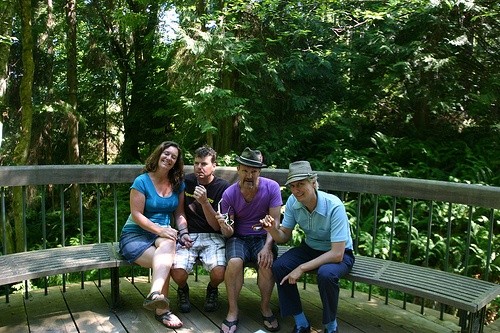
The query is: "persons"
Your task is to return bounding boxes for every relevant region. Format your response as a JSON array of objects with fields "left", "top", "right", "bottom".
[
  {"left": 171, "top": 145, "right": 231, "bottom": 311},
  {"left": 215, "top": 146, "right": 283, "bottom": 333},
  {"left": 259, "top": 161, "right": 356, "bottom": 333},
  {"left": 119, "top": 141, "right": 193, "bottom": 328}
]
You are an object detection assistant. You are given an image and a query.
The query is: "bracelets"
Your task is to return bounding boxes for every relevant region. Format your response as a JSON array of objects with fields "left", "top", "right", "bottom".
[{"left": 178, "top": 227, "right": 189, "bottom": 236}]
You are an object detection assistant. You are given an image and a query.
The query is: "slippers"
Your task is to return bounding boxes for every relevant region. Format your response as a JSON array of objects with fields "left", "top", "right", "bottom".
[
  {"left": 220, "top": 319, "right": 239, "bottom": 333},
  {"left": 143, "top": 292, "right": 170, "bottom": 311},
  {"left": 259, "top": 308, "right": 280, "bottom": 332},
  {"left": 155, "top": 308, "right": 183, "bottom": 329}
]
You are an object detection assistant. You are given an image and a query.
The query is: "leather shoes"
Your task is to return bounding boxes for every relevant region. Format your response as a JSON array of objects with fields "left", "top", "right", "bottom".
[
  {"left": 324, "top": 319, "right": 338, "bottom": 333},
  {"left": 292, "top": 320, "right": 310, "bottom": 333}
]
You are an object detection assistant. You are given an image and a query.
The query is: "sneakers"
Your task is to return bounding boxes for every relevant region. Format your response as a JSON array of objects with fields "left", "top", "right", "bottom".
[
  {"left": 204, "top": 282, "right": 220, "bottom": 312},
  {"left": 176, "top": 284, "right": 191, "bottom": 313}
]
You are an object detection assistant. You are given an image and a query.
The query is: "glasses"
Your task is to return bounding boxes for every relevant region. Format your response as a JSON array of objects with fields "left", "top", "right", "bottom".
[
  {"left": 250, "top": 212, "right": 272, "bottom": 233},
  {"left": 218, "top": 197, "right": 235, "bottom": 227},
  {"left": 177, "top": 227, "right": 199, "bottom": 251}
]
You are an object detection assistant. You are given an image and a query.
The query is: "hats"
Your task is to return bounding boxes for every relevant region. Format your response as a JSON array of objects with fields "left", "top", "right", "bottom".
[
  {"left": 284, "top": 161, "right": 318, "bottom": 185},
  {"left": 236, "top": 147, "right": 267, "bottom": 167}
]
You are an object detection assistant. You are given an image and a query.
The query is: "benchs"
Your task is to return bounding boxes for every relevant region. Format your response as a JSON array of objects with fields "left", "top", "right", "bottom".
[{"left": 0, "top": 165, "right": 499, "bottom": 333}]
